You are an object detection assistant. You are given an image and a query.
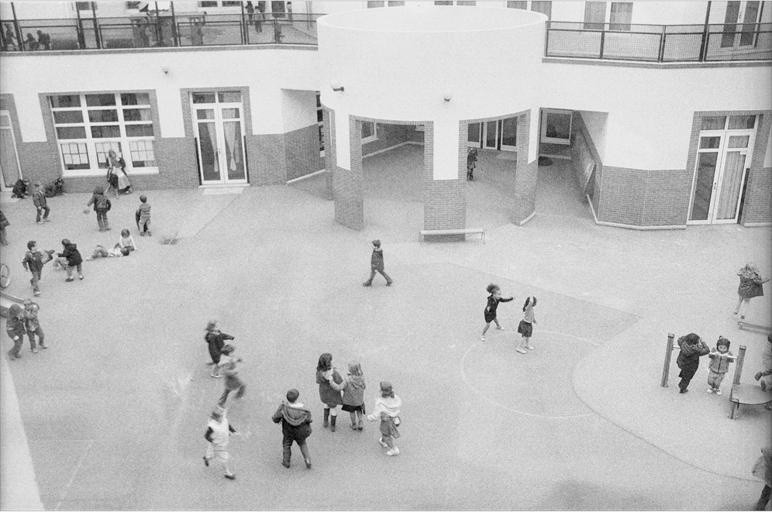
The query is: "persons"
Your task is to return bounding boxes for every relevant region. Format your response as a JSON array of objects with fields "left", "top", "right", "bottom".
[
  {"left": 468, "top": 147, "right": 477, "bottom": 179},
  {"left": 204, "top": 319, "right": 400, "bottom": 479},
  {"left": 516, "top": 297, "right": 538, "bottom": 354},
  {"left": 750, "top": 446, "right": 772, "bottom": 510},
  {"left": 0, "top": 149, "right": 151, "bottom": 361},
  {"left": 755, "top": 331, "right": 771, "bottom": 387},
  {"left": 675, "top": 334, "right": 709, "bottom": 393},
  {"left": 708, "top": 337, "right": 733, "bottom": 395},
  {"left": 4, "top": 21, "right": 49, "bottom": 50},
  {"left": 732, "top": 263, "right": 772, "bottom": 318},
  {"left": 482, "top": 283, "right": 515, "bottom": 339},
  {"left": 362, "top": 240, "right": 393, "bottom": 286},
  {"left": 244, "top": 0, "right": 292, "bottom": 44}
]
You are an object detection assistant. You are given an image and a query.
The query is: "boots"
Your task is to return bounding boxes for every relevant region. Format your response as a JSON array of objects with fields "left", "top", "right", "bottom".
[
  {"left": 323, "top": 407, "right": 330, "bottom": 427},
  {"left": 330, "top": 414, "right": 338, "bottom": 432}
]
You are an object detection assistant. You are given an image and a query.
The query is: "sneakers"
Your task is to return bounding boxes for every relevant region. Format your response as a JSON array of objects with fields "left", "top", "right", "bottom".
[
  {"left": 386, "top": 446, "right": 400, "bottom": 456},
  {"left": 7, "top": 343, "right": 49, "bottom": 360},
  {"left": 378, "top": 437, "right": 388, "bottom": 448},
  {"left": 386, "top": 279, "right": 393, "bottom": 286},
  {"left": 480, "top": 335, "right": 486, "bottom": 342},
  {"left": 30, "top": 278, "right": 41, "bottom": 296},
  {"left": 707, "top": 385, "right": 721, "bottom": 396},
  {"left": 496, "top": 326, "right": 505, "bottom": 330},
  {"left": 281, "top": 462, "right": 290, "bottom": 468},
  {"left": 678, "top": 382, "right": 688, "bottom": 394},
  {"left": 348, "top": 420, "right": 364, "bottom": 431},
  {"left": 304, "top": 458, "right": 312, "bottom": 468},
  {"left": 65, "top": 273, "right": 85, "bottom": 283},
  {"left": 202, "top": 455, "right": 209, "bottom": 467},
  {"left": 223, "top": 472, "right": 235, "bottom": 480},
  {"left": 516, "top": 343, "right": 534, "bottom": 354},
  {"left": 362, "top": 280, "right": 372, "bottom": 286}
]
